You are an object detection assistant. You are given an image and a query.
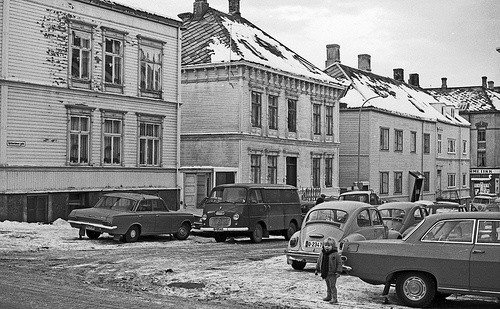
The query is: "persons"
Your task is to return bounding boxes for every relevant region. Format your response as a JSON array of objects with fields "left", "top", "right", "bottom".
[
  {"left": 316, "top": 194, "right": 326, "bottom": 205},
  {"left": 314, "top": 238, "right": 342, "bottom": 304}
]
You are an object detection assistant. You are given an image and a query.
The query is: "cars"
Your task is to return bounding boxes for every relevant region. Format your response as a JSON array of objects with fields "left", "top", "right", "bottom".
[
  {"left": 377, "top": 201, "right": 430, "bottom": 239},
  {"left": 339, "top": 191, "right": 384, "bottom": 205},
  {"left": 286, "top": 201, "right": 390, "bottom": 271},
  {"left": 341, "top": 211, "right": 499, "bottom": 308},
  {"left": 67, "top": 192, "right": 194, "bottom": 243},
  {"left": 414, "top": 193, "right": 500, "bottom": 216}
]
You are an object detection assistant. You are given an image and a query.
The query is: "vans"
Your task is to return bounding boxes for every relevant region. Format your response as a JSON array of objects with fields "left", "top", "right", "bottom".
[{"left": 200, "top": 183, "right": 303, "bottom": 244}]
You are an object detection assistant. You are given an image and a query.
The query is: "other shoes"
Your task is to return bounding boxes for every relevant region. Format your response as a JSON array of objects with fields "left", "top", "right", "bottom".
[
  {"left": 323, "top": 296, "right": 332, "bottom": 301},
  {"left": 329, "top": 297, "right": 338, "bottom": 303}
]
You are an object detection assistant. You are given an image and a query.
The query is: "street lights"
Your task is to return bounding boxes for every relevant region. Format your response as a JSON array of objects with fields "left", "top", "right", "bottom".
[{"left": 357, "top": 94, "right": 389, "bottom": 189}]
